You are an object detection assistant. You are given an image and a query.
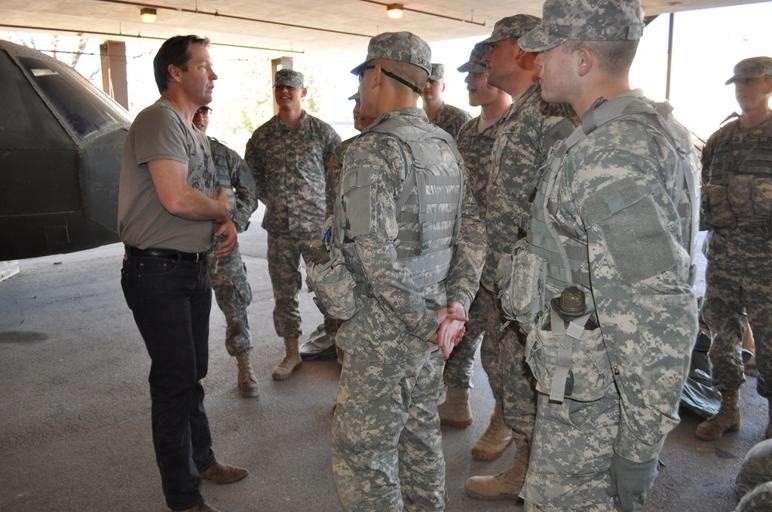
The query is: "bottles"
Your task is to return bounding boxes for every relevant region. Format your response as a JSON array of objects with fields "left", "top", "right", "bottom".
[{"left": 540, "top": 287, "right": 598, "bottom": 335}]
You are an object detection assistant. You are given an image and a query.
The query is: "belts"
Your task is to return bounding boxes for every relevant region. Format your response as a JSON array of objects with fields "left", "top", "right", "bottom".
[{"left": 124, "top": 250, "right": 208, "bottom": 261}]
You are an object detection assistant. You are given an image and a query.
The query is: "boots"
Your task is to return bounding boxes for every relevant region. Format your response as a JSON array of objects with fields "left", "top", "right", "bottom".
[
  {"left": 695, "top": 389, "right": 740, "bottom": 441},
  {"left": 437, "top": 386, "right": 529, "bottom": 503},
  {"left": 272, "top": 336, "right": 302, "bottom": 380},
  {"left": 238, "top": 352, "right": 260, "bottom": 397}
]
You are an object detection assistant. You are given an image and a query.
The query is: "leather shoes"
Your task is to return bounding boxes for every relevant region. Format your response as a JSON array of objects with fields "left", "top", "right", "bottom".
[
  {"left": 179, "top": 503, "right": 221, "bottom": 512},
  {"left": 199, "top": 463, "right": 250, "bottom": 484}
]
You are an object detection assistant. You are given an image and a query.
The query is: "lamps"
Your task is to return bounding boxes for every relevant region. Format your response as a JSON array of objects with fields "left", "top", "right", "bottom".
[
  {"left": 140, "top": 8, "right": 157, "bottom": 22},
  {"left": 387, "top": 4, "right": 403, "bottom": 19}
]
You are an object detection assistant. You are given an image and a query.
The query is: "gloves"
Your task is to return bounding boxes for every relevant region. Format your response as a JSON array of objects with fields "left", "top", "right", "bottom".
[{"left": 606, "top": 453, "right": 660, "bottom": 512}]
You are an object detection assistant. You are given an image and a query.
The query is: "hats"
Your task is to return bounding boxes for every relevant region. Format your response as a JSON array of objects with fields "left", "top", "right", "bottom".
[
  {"left": 725, "top": 58, "right": 772, "bottom": 85},
  {"left": 272, "top": 69, "right": 303, "bottom": 88},
  {"left": 349, "top": 0, "right": 645, "bottom": 99}
]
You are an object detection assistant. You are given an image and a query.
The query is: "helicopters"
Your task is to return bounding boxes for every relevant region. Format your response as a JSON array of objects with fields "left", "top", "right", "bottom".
[{"left": 0, "top": 35, "right": 142, "bottom": 265}]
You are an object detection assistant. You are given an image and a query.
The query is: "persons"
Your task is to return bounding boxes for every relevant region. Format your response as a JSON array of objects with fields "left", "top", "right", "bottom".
[
  {"left": 433, "top": 38, "right": 515, "bottom": 461},
  {"left": 327, "top": 90, "right": 382, "bottom": 221},
  {"left": 326, "top": 33, "right": 486, "bottom": 510},
  {"left": 517, "top": 0, "right": 699, "bottom": 511},
  {"left": 247, "top": 66, "right": 344, "bottom": 381},
  {"left": 191, "top": 102, "right": 261, "bottom": 399},
  {"left": 463, "top": 16, "right": 576, "bottom": 498},
  {"left": 420, "top": 60, "right": 475, "bottom": 140},
  {"left": 115, "top": 37, "right": 252, "bottom": 510},
  {"left": 696, "top": 57, "right": 771, "bottom": 440}
]
